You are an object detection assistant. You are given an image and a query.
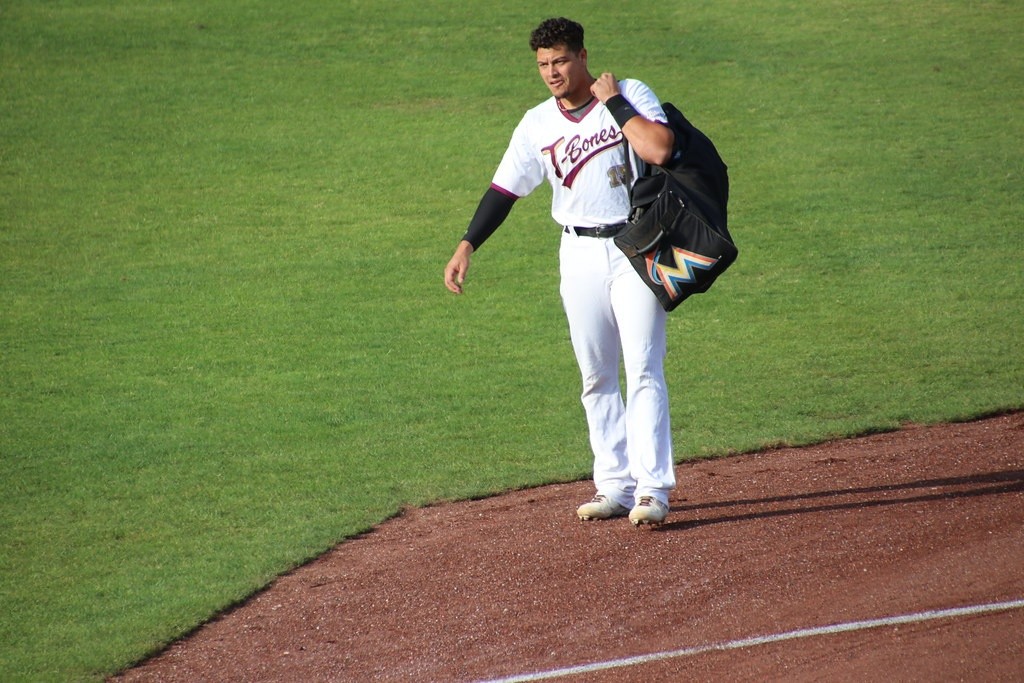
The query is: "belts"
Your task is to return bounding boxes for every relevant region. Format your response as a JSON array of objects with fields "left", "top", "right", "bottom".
[{"left": 564, "top": 223, "right": 626, "bottom": 238}]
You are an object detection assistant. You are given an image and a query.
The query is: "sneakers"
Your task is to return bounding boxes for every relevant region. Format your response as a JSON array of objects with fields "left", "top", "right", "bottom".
[
  {"left": 628, "top": 496, "right": 669, "bottom": 526},
  {"left": 577, "top": 495, "right": 630, "bottom": 520}
]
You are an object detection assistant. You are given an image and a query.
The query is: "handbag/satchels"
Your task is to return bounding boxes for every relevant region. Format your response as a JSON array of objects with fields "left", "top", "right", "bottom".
[{"left": 614, "top": 102, "right": 738, "bottom": 312}]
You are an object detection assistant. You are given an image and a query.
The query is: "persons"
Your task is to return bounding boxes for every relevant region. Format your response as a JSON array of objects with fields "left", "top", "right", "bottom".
[{"left": 444, "top": 16, "right": 675, "bottom": 527}]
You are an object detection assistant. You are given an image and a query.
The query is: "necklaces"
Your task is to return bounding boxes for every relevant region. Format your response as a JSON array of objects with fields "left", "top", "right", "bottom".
[{"left": 557, "top": 96, "right": 591, "bottom": 111}]
